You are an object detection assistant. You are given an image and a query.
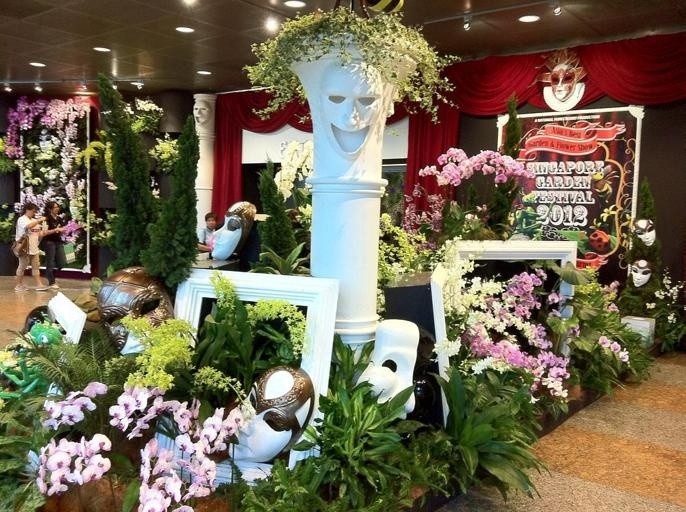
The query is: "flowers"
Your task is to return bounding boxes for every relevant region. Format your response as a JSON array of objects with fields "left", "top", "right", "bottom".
[
  {"left": 274, "top": 138, "right": 315, "bottom": 234},
  {"left": 0, "top": 270, "right": 310, "bottom": 512},
  {"left": 0, "top": 98, "right": 181, "bottom": 270},
  {"left": 376, "top": 147, "right": 536, "bottom": 315},
  {"left": 442, "top": 251, "right": 686, "bottom": 420}
]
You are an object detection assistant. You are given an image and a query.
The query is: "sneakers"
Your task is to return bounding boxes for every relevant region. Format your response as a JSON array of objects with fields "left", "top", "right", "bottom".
[
  {"left": 49, "top": 283, "right": 60, "bottom": 290},
  {"left": 15, "top": 285, "right": 28, "bottom": 291},
  {"left": 36, "top": 284, "right": 49, "bottom": 291}
]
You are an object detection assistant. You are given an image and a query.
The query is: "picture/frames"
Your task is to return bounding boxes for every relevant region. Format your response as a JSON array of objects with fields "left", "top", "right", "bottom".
[
  {"left": 154, "top": 267, "right": 339, "bottom": 486},
  {"left": 498, "top": 105, "right": 644, "bottom": 306},
  {"left": 447, "top": 240, "right": 578, "bottom": 362}
]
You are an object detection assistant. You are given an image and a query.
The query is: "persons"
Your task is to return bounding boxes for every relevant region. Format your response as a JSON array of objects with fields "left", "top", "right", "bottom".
[
  {"left": 197, "top": 213, "right": 216, "bottom": 254},
  {"left": 42, "top": 202, "right": 68, "bottom": 289},
  {"left": 14, "top": 203, "right": 49, "bottom": 291}
]
